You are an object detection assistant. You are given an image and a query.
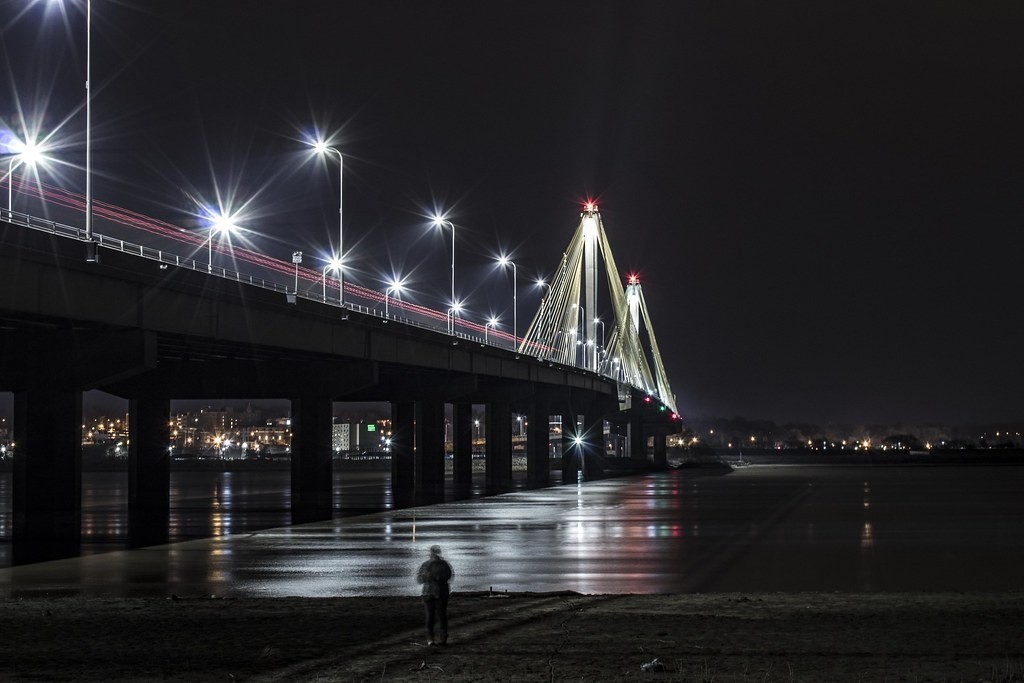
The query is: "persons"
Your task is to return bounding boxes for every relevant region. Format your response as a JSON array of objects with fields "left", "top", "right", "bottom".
[{"left": 418, "top": 545, "right": 452, "bottom": 647}]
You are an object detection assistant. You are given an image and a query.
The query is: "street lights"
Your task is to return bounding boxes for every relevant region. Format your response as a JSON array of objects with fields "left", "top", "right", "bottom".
[
  {"left": 314, "top": 140, "right": 345, "bottom": 306},
  {"left": 447, "top": 303, "right": 460, "bottom": 334},
  {"left": 323, "top": 257, "right": 340, "bottom": 304},
  {"left": 7, "top": 146, "right": 38, "bottom": 221},
  {"left": 434, "top": 215, "right": 456, "bottom": 335},
  {"left": 594, "top": 319, "right": 605, "bottom": 374},
  {"left": 485, "top": 319, "right": 497, "bottom": 344},
  {"left": 572, "top": 304, "right": 585, "bottom": 369},
  {"left": 538, "top": 280, "right": 551, "bottom": 361},
  {"left": 499, "top": 257, "right": 517, "bottom": 351},
  {"left": 385, "top": 281, "right": 402, "bottom": 318},
  {"left": 208, "top": 218, "right": 230, "bottom": 273}
]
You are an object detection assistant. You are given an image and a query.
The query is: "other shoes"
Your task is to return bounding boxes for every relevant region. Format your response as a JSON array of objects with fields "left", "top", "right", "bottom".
[
  {"left": 439, "top": 642, "right": 447, "bottom": 646},
  {"left": 428, "top": 639, "right": 434, "bottom": 647}
]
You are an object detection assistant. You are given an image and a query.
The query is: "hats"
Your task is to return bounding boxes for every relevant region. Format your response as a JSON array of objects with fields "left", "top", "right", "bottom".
[{"left": 430, "top": 544, "right": 441, "bottom": 556}]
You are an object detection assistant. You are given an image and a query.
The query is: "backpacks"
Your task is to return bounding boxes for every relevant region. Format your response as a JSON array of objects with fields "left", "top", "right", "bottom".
[{"left": 427, "top": 557, "right": 451, "bottom": 584}]
